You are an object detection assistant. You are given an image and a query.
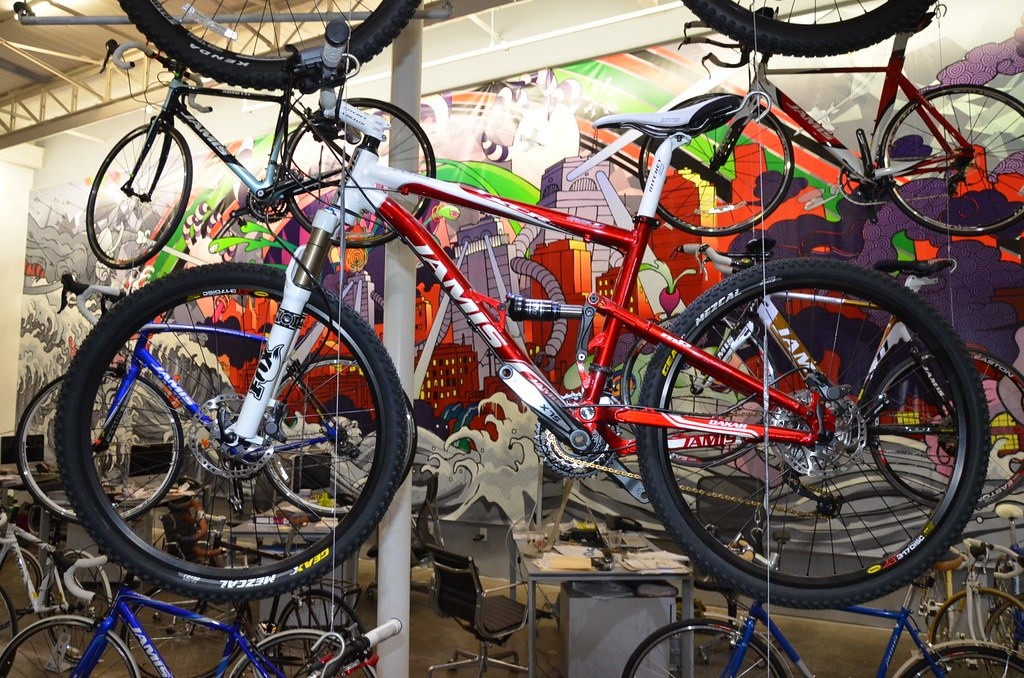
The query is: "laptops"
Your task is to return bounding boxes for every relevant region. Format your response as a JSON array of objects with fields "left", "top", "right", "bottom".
[{"left": 585, "top": 504, "right": 647, "bottom": 548}]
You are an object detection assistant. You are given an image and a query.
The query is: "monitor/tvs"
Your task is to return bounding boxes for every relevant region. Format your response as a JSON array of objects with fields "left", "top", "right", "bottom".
[
  {"left": 126, "top": 443, "right": 173, "bottom": 482},
  {"left": 526, "top": 462, "right": 564, "bottom": 533},
  {"left": 0, "top": 434, "right": 45, "bottom": 465},
  {"left": 290, "top": 453, "right": 331, "bottom": 494}
]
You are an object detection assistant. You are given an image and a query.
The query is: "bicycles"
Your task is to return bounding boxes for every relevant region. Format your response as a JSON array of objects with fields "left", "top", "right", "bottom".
[{"left": 0, "top": 0, "right": 1023, "bottom": 677}]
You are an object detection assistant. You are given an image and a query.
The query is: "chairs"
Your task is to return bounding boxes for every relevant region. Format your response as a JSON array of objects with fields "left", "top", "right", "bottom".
[
  {"left": 652, "top": 477, "right": 791, "bottom": 668},
  {"left": 153, "top": 513, "right": 248, "bottom": 635},
  {"left": 365, "top": 476, "right": 445, "bottom": 601},
  {"left": 425, "top": 542, "right": 553, "bottom": 678}
]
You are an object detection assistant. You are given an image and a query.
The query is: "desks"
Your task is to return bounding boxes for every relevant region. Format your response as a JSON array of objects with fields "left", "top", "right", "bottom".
[
  {"left": 0, "top": 464, "right": 360, "bottom": 629},
  {"left": 510, "top": 523, "right": 694, "bottom": 678}
]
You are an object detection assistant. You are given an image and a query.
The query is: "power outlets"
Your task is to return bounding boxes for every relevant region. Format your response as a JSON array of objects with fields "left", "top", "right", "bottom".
[{"left": 480, "top": 528, "right": 487, "bottom": 542}]
